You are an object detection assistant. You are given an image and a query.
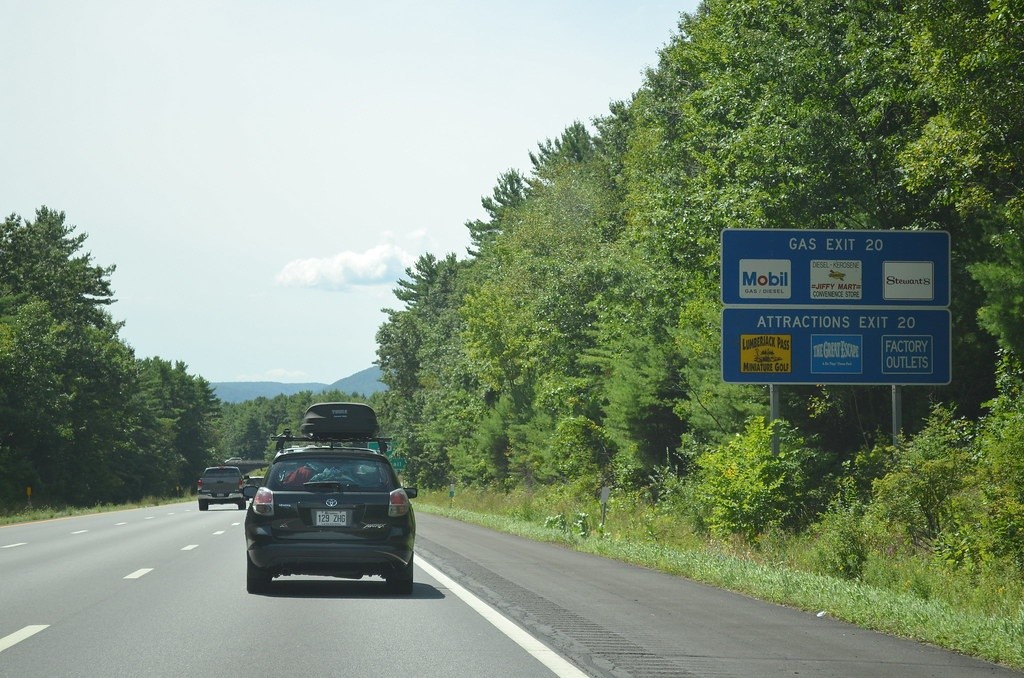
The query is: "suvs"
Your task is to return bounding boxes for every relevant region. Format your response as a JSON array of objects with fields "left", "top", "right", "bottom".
[{"left": 241, "top": 437, "right": 418, "bottom": 595}]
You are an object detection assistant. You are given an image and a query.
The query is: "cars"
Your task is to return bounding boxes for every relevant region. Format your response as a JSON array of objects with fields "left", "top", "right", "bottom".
[
  {"left": 243, "top": 477, "right": 264, "bottom": 501},
  {"left": 225, "top": 457, "right": 243, "bottom": 464}
]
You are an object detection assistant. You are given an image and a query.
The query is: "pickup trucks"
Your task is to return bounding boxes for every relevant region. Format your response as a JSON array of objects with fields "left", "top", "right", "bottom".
[{"left": 196, "top": 466, "right": 246, "bottom": 510}]
